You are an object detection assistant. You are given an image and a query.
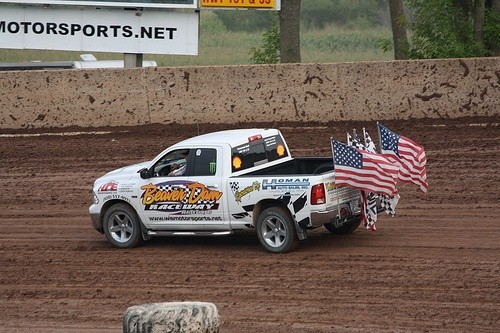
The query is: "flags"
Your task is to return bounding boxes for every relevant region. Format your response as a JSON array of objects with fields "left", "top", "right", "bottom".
[
  {"left": 348, "top": 127, "right": 401, "bottom": 232},
  {"left": 377, "top": 121, "right": 428, "bottom": 193},
  {"left": 331, "top": 137, "right": 401, "bottom": 199}
]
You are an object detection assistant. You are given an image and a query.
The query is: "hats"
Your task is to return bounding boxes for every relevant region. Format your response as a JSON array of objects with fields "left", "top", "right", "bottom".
[{"left": 180, "top": 150, "right": 190, "bottom": 157}]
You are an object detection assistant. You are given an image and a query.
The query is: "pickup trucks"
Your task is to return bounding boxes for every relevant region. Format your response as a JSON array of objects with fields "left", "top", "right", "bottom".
[{"left": 88, "top": 126, "right": 430, "bottom": 253}]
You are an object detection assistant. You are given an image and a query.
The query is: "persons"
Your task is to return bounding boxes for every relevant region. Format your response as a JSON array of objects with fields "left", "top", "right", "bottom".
[{"left": 168, "top": 149, "right": 190, "bottom": 177}]
